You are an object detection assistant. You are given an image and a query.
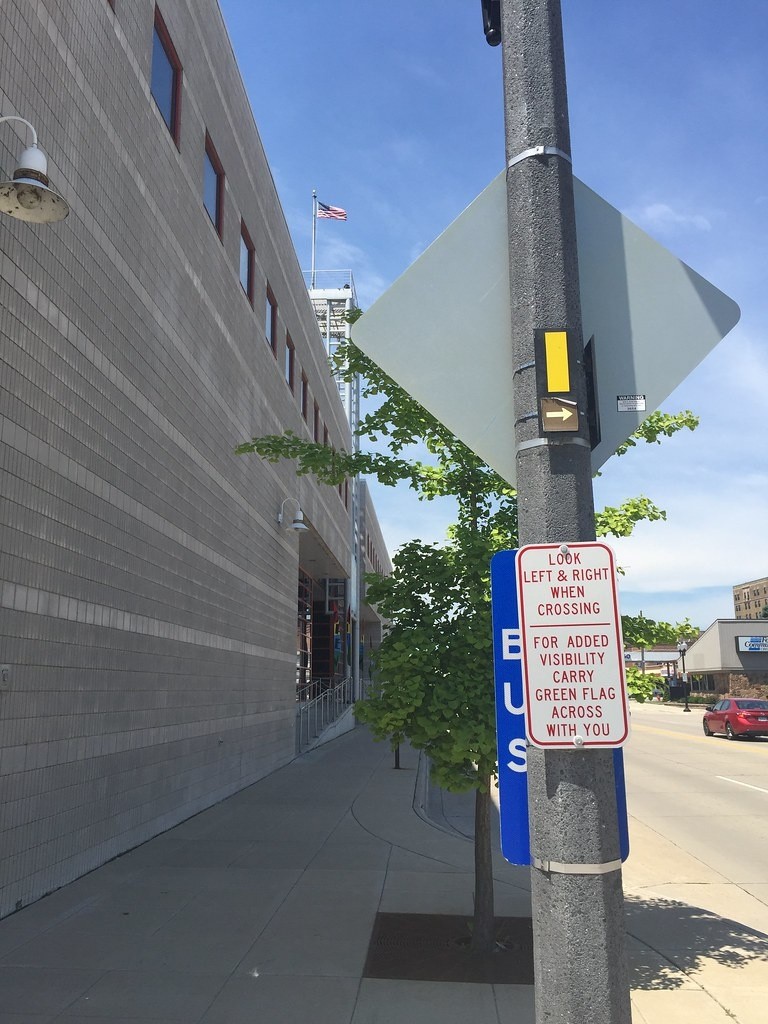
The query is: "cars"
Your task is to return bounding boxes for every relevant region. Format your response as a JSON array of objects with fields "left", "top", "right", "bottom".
[{"left": 702, "top": 698, "right": 768, "bottom": 740}]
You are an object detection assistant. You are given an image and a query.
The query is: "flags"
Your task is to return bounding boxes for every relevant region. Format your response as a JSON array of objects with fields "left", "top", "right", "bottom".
[{"left": 313, "top": 197, "right": 347, "bottom": 221}]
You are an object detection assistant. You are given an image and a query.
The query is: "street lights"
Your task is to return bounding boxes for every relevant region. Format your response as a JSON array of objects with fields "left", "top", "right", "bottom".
[{"left": 677, "top": 639, "right": 691, "bottom": 712}]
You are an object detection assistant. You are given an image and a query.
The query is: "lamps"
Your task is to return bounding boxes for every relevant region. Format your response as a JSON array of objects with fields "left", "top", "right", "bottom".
[
  {"left": 278, "top": 497, "right": 311, "bottom": 533},
  {"left": 0, "top": 115, "right": 71, "bottom": 224}
]
